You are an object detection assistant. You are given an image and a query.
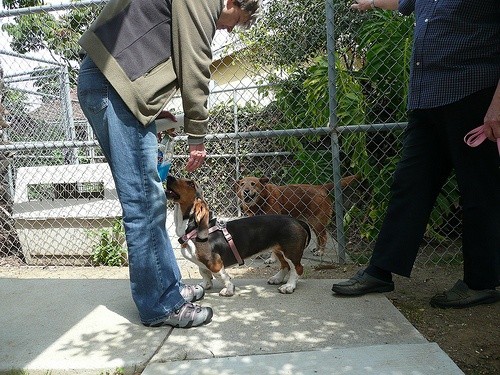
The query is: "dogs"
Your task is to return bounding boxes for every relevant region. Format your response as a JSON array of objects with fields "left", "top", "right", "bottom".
[
  {"left": 164, "top": 174, "right": 312, "bottom": 298},
  {"left": 234, "top": 172, "right": 358, "bottom": 257},
  {"left": 203, "top": 208, "right": 209, "bottom": 211}
]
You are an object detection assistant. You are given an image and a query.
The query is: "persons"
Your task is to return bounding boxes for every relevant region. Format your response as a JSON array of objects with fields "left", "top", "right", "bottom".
[
  {"left": 331, "top": 0, "right": 500, "bottom": 309},
  {"left": 77, "top": 0, "right": 264, "bottom": 329}
]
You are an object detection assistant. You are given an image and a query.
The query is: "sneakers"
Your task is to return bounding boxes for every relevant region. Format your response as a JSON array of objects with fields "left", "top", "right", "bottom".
[
  {"left": 429, "top": 279, "right": 500, "bottom": 309},
  {"left": 143, "top": 300, "right": 213, "bottom": 328},
  {"left": 176, "top": 283, "right": 206, "bottom": 303},
  {"left": 332, "top": 269, "right": 394, "bottom": 295}
]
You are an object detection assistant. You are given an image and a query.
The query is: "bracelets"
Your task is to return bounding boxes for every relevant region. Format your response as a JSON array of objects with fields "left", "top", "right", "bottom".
[{"left": 371, "top": 0, "right": 378, "bottom": 11}]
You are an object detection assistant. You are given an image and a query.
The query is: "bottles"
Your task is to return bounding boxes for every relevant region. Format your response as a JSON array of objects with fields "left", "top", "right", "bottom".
[{"left": 157, "top": 126, "right": 174, "bottom": 181}]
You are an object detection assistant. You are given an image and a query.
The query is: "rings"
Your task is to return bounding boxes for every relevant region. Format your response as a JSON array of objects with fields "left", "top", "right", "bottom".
[{"left": 195, "top": 160, "right": 199, "bottom": 164}]
